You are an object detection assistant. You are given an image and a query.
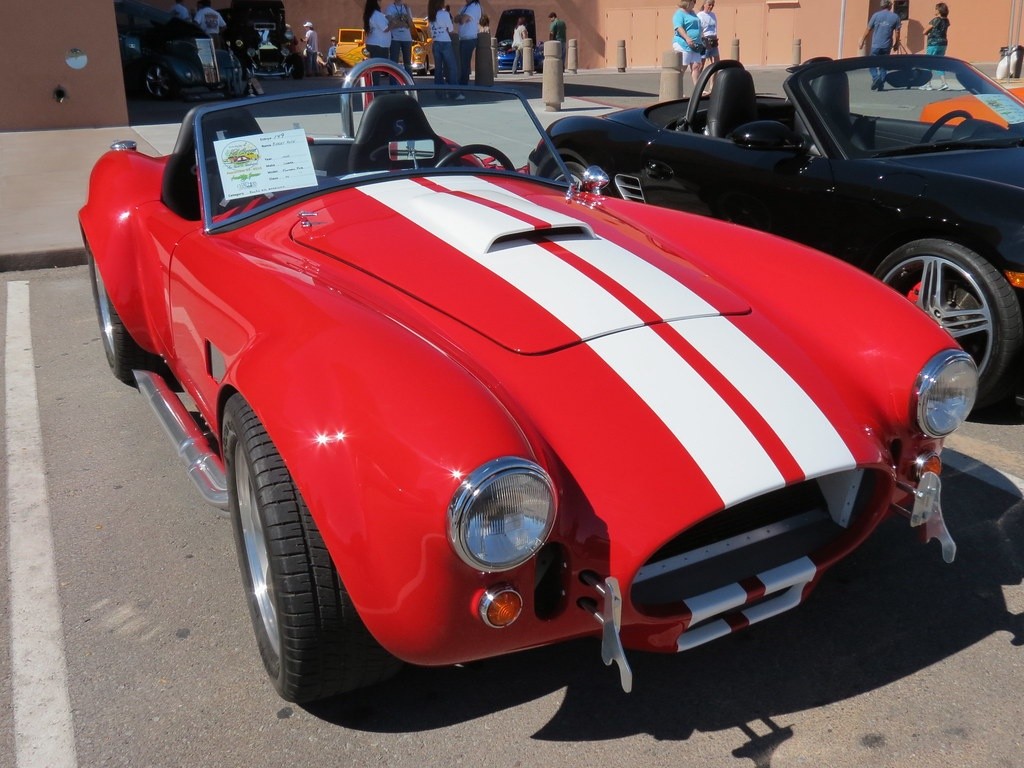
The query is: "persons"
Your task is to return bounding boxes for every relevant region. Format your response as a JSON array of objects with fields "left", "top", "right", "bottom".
[
  {"left": 282, "top": 21, "right": 323, "bottom": 77},
  {"left": 326, "top": 36, "right": 345, "bottom": 76},
  {"left": 672, "top": 0, "right": 720, "bottom": 98},
  {"left": 548, "top": 12, "right": 567, "bottom": 72},
  {"left": 511, "top": 16, "right": 538, "bottom": 75},
  {"left": 169, "top": 0, "right": 228, "bottom": 50},
  {"left": 362, "top": 0, "right": 421, "bottom": 93},
  {"left": 858, "top": 0, "right": 902, "bottom": 92},
  {"left": 922, "top": 3, "right": 950, "bottom": 92},
  {"left": 426, "top": 0, "right": 482, "bottom": 101}
]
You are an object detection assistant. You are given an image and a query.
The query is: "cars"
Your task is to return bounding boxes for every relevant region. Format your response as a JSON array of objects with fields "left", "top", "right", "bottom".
[
  {"left": 114, "top": 0, "right": 244, "bottom": 100},
  {"left": 331, "top": 16, "right": 434, "bottom": 75}
]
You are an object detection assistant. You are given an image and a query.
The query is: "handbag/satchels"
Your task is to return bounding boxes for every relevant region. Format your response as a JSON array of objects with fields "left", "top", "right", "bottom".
[
  {"left": 694, "top": 43, "right": 704, "bottom": 52},
  {"left": 707, "top": 38, "right": 718, "bottom": 48}
]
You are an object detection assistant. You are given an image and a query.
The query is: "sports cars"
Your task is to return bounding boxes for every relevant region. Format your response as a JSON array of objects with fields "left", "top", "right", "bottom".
[
  {"left": 527, "top": 54, "right": 1024, "bottom": 422},
  {"left": 77, "top": 56, "right": 979, "bottom": 704},
  {"left": 498, "top": 39, "right": 544, "bottom": 73}
]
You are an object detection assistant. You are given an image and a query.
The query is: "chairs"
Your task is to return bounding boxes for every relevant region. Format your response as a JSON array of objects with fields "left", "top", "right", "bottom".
[
  {"left": 161, "top": 102, "right": 264, "bottom": 221},
  {"left": 705, "top": 67, "right": 760, "bottom": 139},
  {"left": 330, "top": 92, "right": 480, "bottom": 180},
  {"left": 794, "top": 56, "right": 851, "bottom": 149}
]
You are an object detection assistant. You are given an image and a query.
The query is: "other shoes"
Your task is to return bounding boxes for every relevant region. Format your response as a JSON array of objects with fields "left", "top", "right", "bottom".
[
  {"left": 871, "top": 78, "right": 881, "bottom": 90},
  {"left": 926, "top": 85, "right": 932, "bottom": 90},
  {"left": 878, "top": 86, "right": 883, "bottom": 91},
  {"left": 455, "top": 95, "right": 466, "bottom": 100},
  {"left": 702, "top": 90, "right": 712, "bottom": 96},
  {"left": 939, "top": 84, "right": 949, "bottom": 91},
  {"left": 442, "top": 93, "right": 450, "bottom": 98}
]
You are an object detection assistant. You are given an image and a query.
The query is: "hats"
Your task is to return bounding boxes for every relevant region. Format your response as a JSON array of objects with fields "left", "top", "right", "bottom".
[
  {"left": 303, "top": 22, "right": 313, "bottom": 27},
  {"left": 331, "top": 36, "right": 338, "bottom": 42}
]
